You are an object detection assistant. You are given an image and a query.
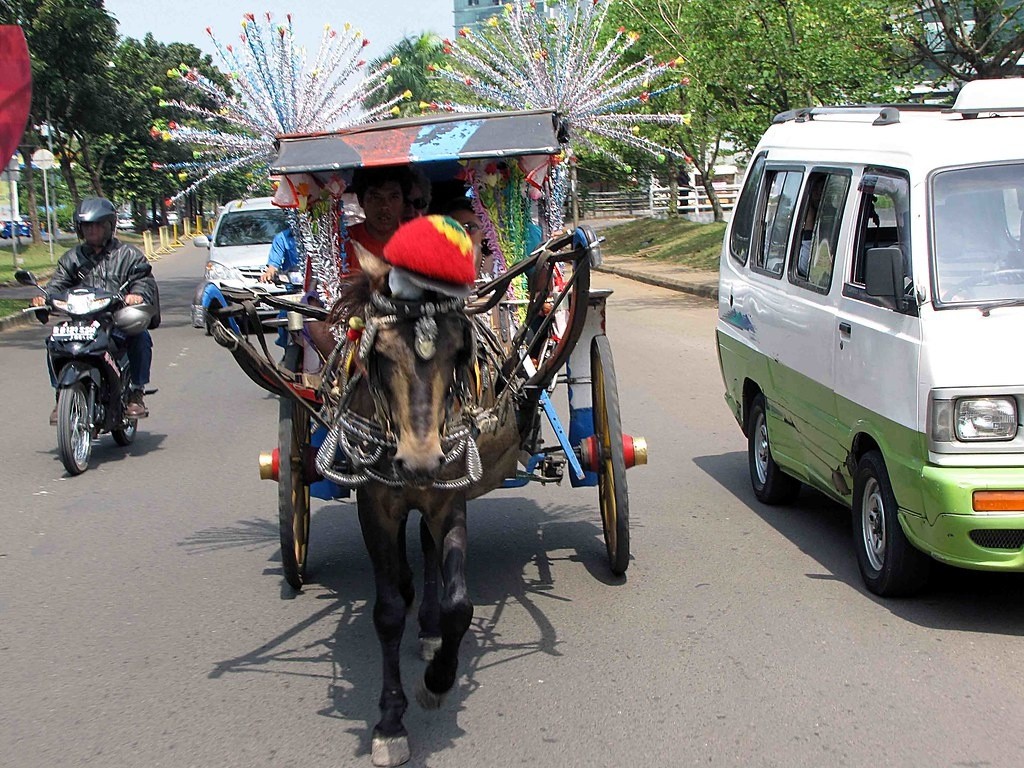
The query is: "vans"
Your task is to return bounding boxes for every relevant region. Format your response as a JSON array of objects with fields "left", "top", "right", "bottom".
[
  {"left": 716, "top": 75, "right": 1024, "bottom": 602},
  {"left": 192, "top": 196, "right": 304, "bottom": 328}
]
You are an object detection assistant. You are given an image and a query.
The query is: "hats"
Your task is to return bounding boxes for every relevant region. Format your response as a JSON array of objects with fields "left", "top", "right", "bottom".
[{"left": 428, "top": 179, "right": 475, "bottom": 214}]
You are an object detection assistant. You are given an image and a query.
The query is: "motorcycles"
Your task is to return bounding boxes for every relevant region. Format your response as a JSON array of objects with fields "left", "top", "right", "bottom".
[{"left": 13, "top": 271, "right": 159, "bottom": 475}]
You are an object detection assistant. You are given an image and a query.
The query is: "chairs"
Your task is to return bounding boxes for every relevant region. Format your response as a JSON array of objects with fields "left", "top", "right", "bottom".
[{"left": 941, "top": 177, "right": 1021, "bottom": 262}]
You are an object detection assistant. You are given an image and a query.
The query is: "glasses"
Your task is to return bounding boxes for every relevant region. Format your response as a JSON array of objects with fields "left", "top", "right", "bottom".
[
  {"left": 462, "top": 222, "right": 483, "bottom": 235},
  {"left": 403, "top": 197, "right": 427, "bottom": 209}
]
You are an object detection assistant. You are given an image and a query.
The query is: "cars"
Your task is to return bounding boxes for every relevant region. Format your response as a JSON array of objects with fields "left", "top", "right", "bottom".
[{"left": 117, "top": 209, "right": 222, "bottom": 232}]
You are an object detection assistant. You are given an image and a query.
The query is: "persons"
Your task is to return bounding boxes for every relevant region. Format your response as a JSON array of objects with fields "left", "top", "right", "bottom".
[
  {"left": 299, "top": 162, "right": 498, "bottom": 395},
  {"left": 259, "top": 225, "right": 303, "bottom": 369},
  {"left": 797, "top": 171, "right": 841, "bottom": 276},
  {"left": 676, "top": 165, "right": 690, "bottom": 214},
  {"left": 530, "top": 301, "right": 561, "bottom": 369},
  {"left": 626, "top": 169, "right": 669, "bottom": 207},
  {"left": 32, "top": 195, "right": 161, "bottom": 426}
]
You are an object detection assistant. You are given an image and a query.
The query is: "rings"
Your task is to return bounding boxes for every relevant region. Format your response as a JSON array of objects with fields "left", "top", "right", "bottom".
[
  {"left": 35, "top": 304, "right": 38, "bottom": 306},
  {"left": 135, "top": 301, "right": 138, "bottom": 304}
]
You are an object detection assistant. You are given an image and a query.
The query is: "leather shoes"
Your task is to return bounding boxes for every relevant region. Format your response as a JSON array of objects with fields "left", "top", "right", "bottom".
[
  {"left": 50, "top": 392, "right": 60, "bottom": 425},
  {"left": 124, "top": 386, "right": 146, "bottom": 418}
]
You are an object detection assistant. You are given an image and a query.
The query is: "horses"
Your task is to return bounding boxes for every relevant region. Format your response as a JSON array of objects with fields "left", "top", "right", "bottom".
[{"left": 332, "top": 239, "right": 521, "bottom": 767}]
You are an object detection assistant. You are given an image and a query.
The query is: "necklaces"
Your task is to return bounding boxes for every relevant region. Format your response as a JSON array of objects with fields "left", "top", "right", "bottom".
[{"left": 475, "top": 253, "right": 486, "bottom": 288}]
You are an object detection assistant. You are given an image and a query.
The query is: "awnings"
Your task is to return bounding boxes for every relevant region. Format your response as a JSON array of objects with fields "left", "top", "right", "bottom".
[
  {"left": 694, "top": 166, "right": 737, "bottom": 175},
  {"left": 0, "top": 25, "right": 31, "bottom": 174}
]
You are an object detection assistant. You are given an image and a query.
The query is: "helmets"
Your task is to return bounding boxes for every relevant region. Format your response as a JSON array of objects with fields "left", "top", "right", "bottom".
[
  {"left": 112, "top": 303, "right": 157, "bottom": 335},
  {"left": 73, "top": 196, "right": 117, "bottom": 240}
]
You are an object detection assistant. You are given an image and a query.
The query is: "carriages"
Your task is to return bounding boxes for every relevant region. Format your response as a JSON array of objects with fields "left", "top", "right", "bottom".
[{"left": 190, "top": 107, "right": 647, "bottom": 767}]
